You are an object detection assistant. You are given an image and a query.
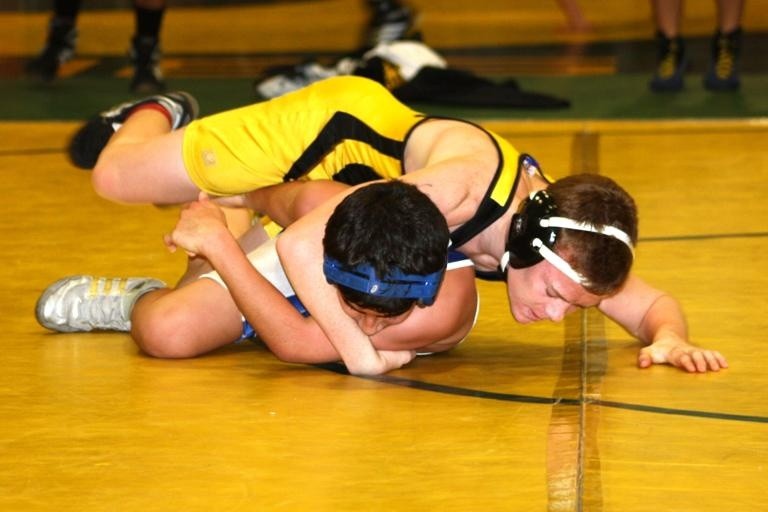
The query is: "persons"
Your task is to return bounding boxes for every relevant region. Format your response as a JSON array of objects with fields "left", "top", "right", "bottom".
[
  {"left": 254, "top": 1, "right": 568, "bottom": 111},
  {"left": 28, "top": 2, "right": 168, "bottom": 93},
  {"left": 651, "top": 0, "right": 746, "bottom": 93},
  {"left": 31, "top": 178, "right": 478, "bottom": 368},
  {"left": 65, "top": 74, "right": 727, "bottom": 377}
]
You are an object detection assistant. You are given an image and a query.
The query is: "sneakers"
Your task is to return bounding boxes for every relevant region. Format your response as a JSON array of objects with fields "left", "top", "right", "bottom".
[
  {"left": 130, "top": 36, "right": 165, "bottom": 93},
  {"left": 706, "top": 27, "right": 740, "bottom": 91},
  {"left": 650, "top": 33, "right": 682, "bottom": 91},
  {"left": 34, "top": 277, "right": 166, "bottom": 334},
  {"left": 69, "top": 92, "right": 199, "bottom": 169},
  {"left": 26, "top": 18, "right": 76, "bottom": 77}
]
